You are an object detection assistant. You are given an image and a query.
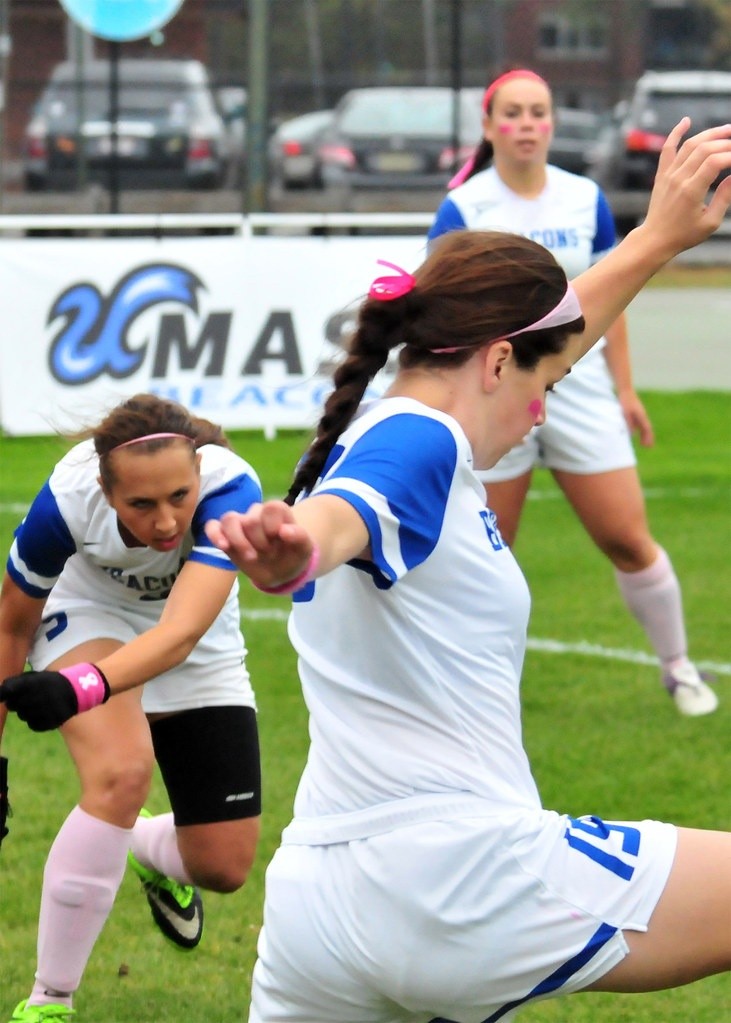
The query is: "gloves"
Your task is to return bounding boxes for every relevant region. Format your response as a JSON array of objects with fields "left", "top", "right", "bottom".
[
  {"left": 0, "top": 754, "right": 9, "bottom": 843},
  {"left": 0, "top": 661, "right": 112, "bottom": 733}
]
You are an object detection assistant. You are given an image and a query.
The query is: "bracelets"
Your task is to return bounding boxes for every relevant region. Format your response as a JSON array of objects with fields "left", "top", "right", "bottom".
[{"left": 249, "top": 534, "right": 316, "bottom": 599}]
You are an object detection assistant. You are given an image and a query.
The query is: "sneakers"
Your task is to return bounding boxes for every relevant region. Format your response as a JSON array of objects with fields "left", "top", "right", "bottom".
[
  {"left": 7, "top": 998, "right": 71, "bottom": 1023},
  {"left": 127, "top": 808, "right": 203, "bottom": 954},
  {"left": 666, "top": 660, "right": 718, "bottom": 718}
]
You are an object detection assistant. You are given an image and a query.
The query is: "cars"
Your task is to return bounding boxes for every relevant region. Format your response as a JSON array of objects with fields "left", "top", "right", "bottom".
[
  {"left": 23, "top": 59, "right": 225, "bottom": 190},
  {"left": 226, "top": 86, "right": 609, "bottom": 191},
  {"left": 613, "top": 71, "right": 731, "bottom": 189}
]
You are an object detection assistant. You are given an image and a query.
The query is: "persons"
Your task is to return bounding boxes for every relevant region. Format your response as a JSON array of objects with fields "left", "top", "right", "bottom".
[
  {"left": 202, "top": 113, "right": 729, "bottom": 1023},
  {"left": 420, "top": 69, "right": 721, "bottom": 718},
  {"left": 1, "top": 385, "right": 265, "bottom": 1021}
]
